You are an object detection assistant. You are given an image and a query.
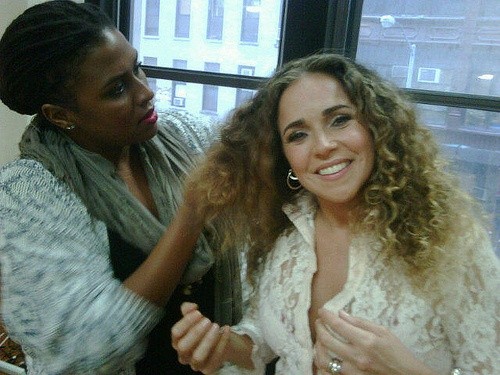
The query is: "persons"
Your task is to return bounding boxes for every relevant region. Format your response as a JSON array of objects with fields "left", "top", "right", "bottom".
[
  {"left": 0, "top": 1, "right": 253, "bottom": 375},
  {"left": 172, "top": 53, "right": 500, "bottom": 375}
]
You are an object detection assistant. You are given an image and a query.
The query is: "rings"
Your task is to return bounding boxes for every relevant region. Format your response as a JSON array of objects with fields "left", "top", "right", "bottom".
[{"left": 327, "top": 359, "right": 342, "bottom": 375}]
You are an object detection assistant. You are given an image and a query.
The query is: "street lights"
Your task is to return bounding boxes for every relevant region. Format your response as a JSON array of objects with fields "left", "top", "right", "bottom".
[{"left": 380, "top": 15, "right": 417, "bottom": 90}]
